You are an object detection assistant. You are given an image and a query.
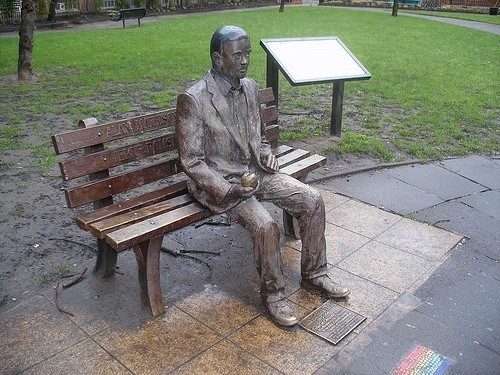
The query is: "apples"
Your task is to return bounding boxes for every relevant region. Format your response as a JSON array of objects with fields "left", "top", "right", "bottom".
[{"left": 241, "top": 172, "right": 258, "bottom": 187}]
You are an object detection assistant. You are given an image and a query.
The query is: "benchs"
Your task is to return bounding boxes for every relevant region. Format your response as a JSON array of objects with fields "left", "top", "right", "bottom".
[
  {"left": 52, "top": 87, "right": 327, "bottom": 316},
  {"left": 115, "top": 8, "right": 145, "bottom": 28}
]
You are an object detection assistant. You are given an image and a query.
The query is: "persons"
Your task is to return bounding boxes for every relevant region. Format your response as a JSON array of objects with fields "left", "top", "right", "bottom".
[{"left": 176, "top": 25, "right": 353, "bottom": 327}]
